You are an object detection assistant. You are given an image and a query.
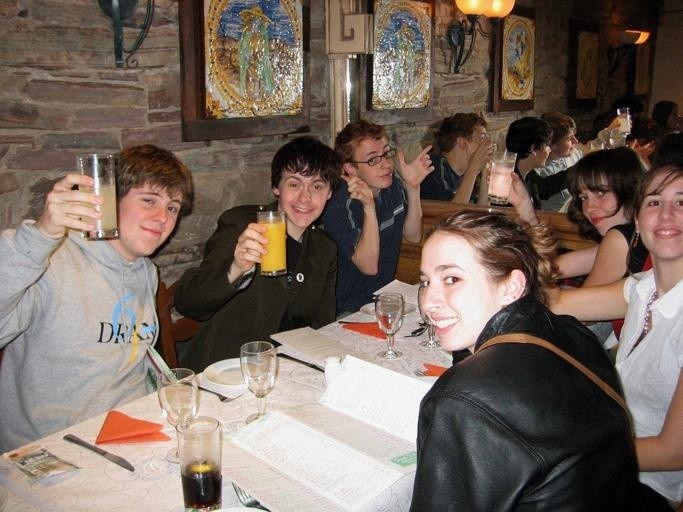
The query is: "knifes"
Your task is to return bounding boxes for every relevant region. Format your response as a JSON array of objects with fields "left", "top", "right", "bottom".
[
  {"left": 64, "top": 434, "right": 134, "bottom": 471},
  {"left": 278, "top": 352, "right": 327, "bottom": 373}
]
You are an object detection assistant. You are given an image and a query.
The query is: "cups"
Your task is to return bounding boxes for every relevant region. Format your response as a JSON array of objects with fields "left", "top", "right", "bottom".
[
  {"left": 487, "top": 150, "right": 517, "bottom": 204},
  {"left": 610, "top": 129, "right": 625, "bottom": 147},
  {"left": 617, "top": 107, "right": 632, "bottom": 135},
  {"left": 76, "top": 153, "right": 120, "bottom": 241},
  {"left": 174, "top": 415, "right": 223, "bottom": 511},
  {"left": 258, "top": 211, "right": 288, "bottom": 276},
  {"left": 589, "top": 140, "right": 605, "bottom": 152}
]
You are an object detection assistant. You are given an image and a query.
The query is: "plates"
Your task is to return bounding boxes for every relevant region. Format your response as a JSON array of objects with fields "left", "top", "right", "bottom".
[
  {"left": 204, "top": 358, "right": 261, "bottom": 385},
  {"left": 361, "top": 302, "right": 417, "bottom": 318}
]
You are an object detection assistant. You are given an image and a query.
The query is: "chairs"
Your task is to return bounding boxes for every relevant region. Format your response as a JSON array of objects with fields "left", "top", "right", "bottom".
[{"left": 155, "top": 272, "right": 214, "bottom": 374}]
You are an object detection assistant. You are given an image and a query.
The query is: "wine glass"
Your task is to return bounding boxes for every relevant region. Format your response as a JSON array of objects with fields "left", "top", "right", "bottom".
[
  {"left": 417, "top": 287, "right": 442, "bottom": 349},
  {"left": 375, "top": 293, "right": 405, "bottom": 358},
  {"left": 156, "top": 368, "right": 201, "bottom": 463},
  {"left": 240, "top": 340, "right": 277, "bottom": 425}
]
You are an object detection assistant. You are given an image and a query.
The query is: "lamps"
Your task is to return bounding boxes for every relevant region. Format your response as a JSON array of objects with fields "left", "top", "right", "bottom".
[{"left": 443, "top": 0, "right": 518, "bottom": 75}]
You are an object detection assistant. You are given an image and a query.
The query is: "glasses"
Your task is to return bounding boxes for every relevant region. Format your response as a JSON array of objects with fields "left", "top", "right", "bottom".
[{"left": 352, "top": 148, "right": 398, "bottom": 168}]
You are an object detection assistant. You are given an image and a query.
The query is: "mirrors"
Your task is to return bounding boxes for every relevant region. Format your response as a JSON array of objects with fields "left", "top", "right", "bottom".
[{"left": 322, "top": 0, "right": 682, "bottom": 218}]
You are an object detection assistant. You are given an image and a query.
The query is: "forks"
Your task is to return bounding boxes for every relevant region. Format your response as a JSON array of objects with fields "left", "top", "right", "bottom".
[
  {"left": 232, "top": 483, "right": 273, "bottom": 511},
  {"left": 181, "top": 380, "right": 244, "bottom": 402}
]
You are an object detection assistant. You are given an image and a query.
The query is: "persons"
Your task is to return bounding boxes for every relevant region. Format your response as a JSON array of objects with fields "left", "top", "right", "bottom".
[
  {"left": 530, "top": 160, "right": 681, "bottom": 512},
  {"left": 0, "top": 142, "right": 197, "bottom": 451},
  {"left": 420, "top": 92, "right": 683, "bottom": 211},
  {"left": 172, "top": 134, "right": 339, "bottom": 376},
  {"left": 408, "top": 204, "right": 641, "bottom": 511},
  {"left": 534, "top": 146, "right": 653, "bottom": 346},
  {"left": 334, "top": 118, "right": 436, "bottom": 322}
]
